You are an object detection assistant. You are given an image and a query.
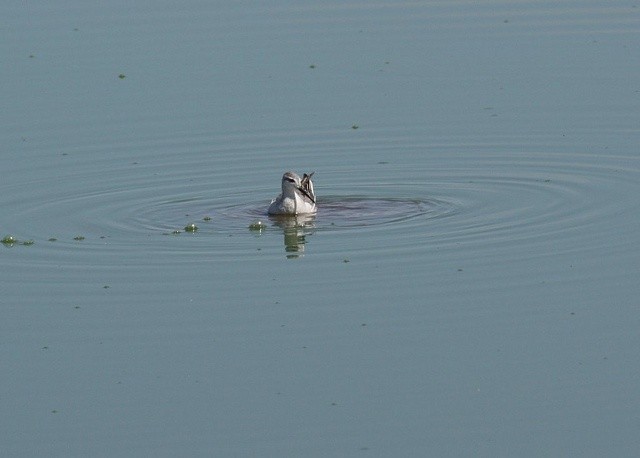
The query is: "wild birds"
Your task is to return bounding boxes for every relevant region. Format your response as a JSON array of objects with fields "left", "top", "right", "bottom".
[{"left": 267, "top": 171, "right": 319, "bottom": 215}]
